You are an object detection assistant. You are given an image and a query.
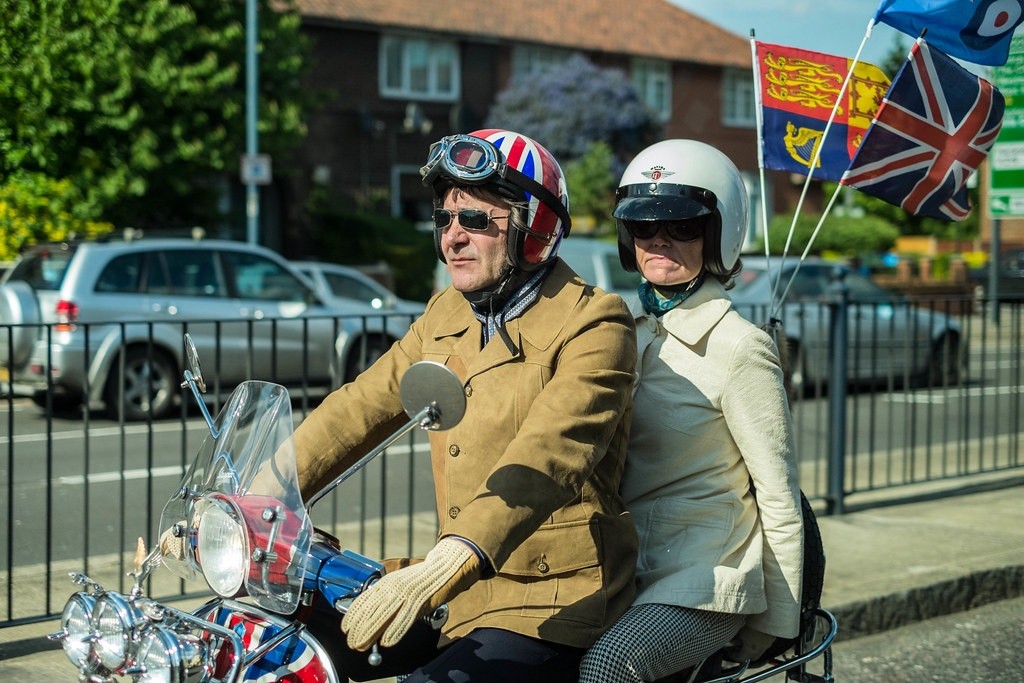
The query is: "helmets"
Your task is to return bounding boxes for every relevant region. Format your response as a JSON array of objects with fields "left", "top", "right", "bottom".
[
  {"left": 612, "top": 139, "right": 749, "bottom": 285},
  {"left": 419, "top": 129, "right": 572, "bottom": 356}
]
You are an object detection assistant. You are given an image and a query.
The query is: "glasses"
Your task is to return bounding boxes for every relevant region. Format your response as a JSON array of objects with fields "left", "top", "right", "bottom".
[
  {"left": 432, "top": 208, "right": 509, "bottom": 231},
  {"left": 630, "top": 218, "right": 705, "bottom": 242}
]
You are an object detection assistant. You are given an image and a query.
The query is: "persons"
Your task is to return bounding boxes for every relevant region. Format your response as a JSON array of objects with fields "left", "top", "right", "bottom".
[
  {"left": 585, "top": 138, "right": 805, "bottom": 683},
  {"left": 159, "top": 128, "right": 638, "bottom": 683}
]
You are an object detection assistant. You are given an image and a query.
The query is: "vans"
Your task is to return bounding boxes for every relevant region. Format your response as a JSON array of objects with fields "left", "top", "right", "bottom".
[{"left": 439, "top": 244, "right": 650, "bottom": 317}]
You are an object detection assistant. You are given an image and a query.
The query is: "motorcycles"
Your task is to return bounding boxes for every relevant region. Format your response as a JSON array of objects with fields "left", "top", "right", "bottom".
[
  {"left": 53, "top": 332, "right": 841, "bottom": 683},
  {"left": 0, "top": 236, "right": 400, "bottom": 419}
]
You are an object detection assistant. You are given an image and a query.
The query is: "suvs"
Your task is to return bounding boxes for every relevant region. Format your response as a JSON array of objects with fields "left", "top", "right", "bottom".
[{"left": 232, "top": 263, "right": 427, "bottom": 324}]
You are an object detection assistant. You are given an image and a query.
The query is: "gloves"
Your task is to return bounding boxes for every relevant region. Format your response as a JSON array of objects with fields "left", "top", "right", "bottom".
[
  {"left": 341, "top": 536, "right": 482, "bottom": 652},
  {"left": 160, "top": 509, "right": 201, "bottom": 561},
  {"left": 731, "top": 626, "right": 776, "bottom": 663}
]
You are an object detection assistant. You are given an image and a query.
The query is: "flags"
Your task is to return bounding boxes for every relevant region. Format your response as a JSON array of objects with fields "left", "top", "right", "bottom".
[
  {"left": 872, "top": 0, "right": 1024, "bottom": 67},
  {"left": 840, "top": 38, "right": 1005, "bottom": 222},
  {"left": 745, "top": 41, "right": 892, "bottom": 182}
]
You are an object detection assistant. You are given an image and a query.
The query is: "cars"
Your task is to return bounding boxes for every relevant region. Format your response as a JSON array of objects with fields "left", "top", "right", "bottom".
[{"left": 726, "top": 257, "right": 971, "bottom": 394}]
